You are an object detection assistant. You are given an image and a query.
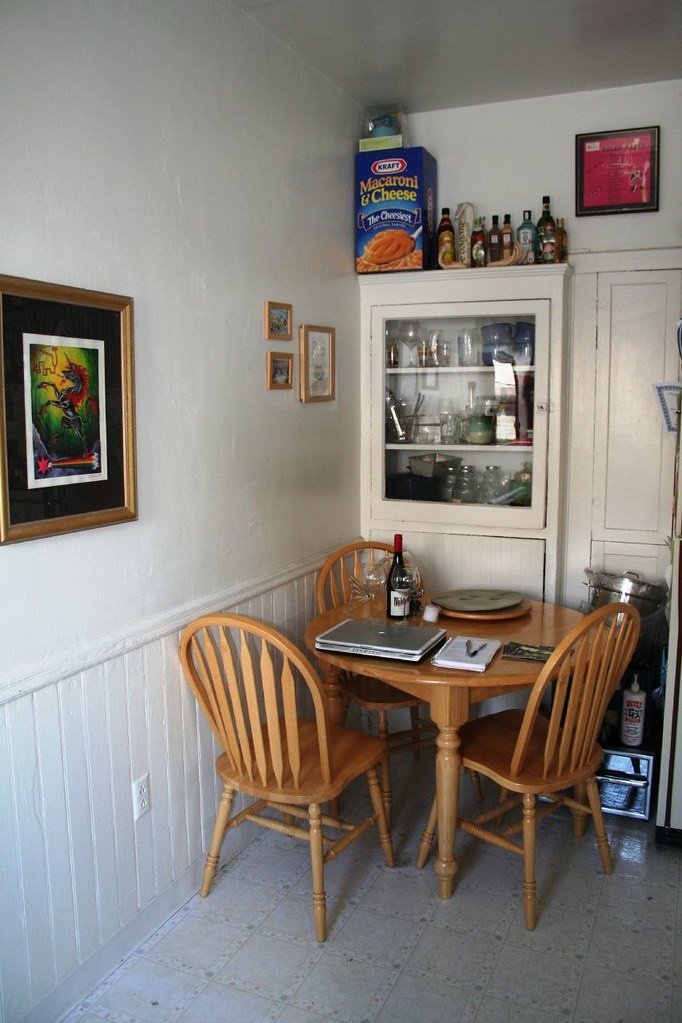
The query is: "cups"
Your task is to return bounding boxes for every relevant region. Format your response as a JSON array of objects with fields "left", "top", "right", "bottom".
[
  {"left": 426, "top": 329, "right": 449, "bottom": 365},
  {"left": 440, "top": 412, "right": 462, "bottom": 444},
  {"left": 457, "top": 328, "right": 480, "bottom": 367},
  {"left": 481, "top": 320, "right": 536, "bottom": 365}
]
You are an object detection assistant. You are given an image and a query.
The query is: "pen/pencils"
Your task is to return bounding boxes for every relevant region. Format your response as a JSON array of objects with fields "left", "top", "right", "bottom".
[
  {"left": 470, "top": 643, "right": 487, "bottom": 657},
  {"left": 466, "top": 640, "right": 471, "bottom": 656}
]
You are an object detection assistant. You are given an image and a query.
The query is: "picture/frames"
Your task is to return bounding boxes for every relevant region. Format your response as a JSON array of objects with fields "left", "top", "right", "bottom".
[
  {"left": 1, "top": 274, "right": 137, "bottom": 545},
  {"left": 266, "top": 352, "right": 294, "bottom": 389},
  {"left": 574, "top": 126, "right": 658, "bottom": 216},
  {"left": 300, "top": 324, "right": 336, "bottom": 402},
  {"left": 265, "top": 301, "right": 293, "bottom": 341}
]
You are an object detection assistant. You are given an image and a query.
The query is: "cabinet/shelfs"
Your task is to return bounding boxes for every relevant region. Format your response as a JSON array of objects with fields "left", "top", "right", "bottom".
[{"left": 359, "top": 261, "right": 573, "bottom": 605}]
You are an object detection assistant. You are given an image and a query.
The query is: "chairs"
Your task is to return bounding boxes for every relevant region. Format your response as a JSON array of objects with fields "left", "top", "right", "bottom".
[
  {"left": 178, "top": 613, "right": 396, "bottom": 943},
  {"left": 417, "top": 604, "right": 642, "bottom": 926},
  {"left": 315, "top": 542, "right": 483, "bottom": 832}
]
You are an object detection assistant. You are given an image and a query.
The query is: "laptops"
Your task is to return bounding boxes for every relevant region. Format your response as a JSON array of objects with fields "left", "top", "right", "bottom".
[{"left": 314, "top": 618, "right": 448, "bottom": 663}]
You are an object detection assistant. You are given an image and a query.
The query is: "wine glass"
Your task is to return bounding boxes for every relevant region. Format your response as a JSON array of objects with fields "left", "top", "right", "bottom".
[
  {"left": 386, "top": 319, "right": 402, "bottom": 368},
  {"left": 399, "top": 321, "right": 422, "bottom": 368},
  {"left": 391, "top": 563, "right": 421, "bottom": 626}
]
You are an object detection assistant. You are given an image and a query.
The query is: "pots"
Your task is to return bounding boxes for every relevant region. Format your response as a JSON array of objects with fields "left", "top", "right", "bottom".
[{"left": 583, "top": 565, "right": 670, "bottom": 625}]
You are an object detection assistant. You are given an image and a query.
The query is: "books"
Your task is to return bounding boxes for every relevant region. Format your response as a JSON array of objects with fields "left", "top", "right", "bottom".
[{"left": 431, "top": 636, "right": 503, "bottom": 673}]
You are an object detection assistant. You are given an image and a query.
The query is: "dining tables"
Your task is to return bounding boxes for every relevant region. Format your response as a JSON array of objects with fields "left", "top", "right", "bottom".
[{"left": 306, "top": 588, "right": 618, "bottom": 868}]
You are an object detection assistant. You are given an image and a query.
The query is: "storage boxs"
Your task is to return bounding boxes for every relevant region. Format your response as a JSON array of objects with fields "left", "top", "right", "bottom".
[{"left": 353, "top": 146, "right": 437, "bottom": 274}]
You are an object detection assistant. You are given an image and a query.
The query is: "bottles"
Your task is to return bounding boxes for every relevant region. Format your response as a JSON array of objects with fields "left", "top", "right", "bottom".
[
  {"left": 437, "top": 196, "right": 567, "bottom": 270},
  {"left": 387, "top": 533, "right": 410, "bottom": 621},
  {"left": 480, "top": 465, "right": 503, "bottom": 504},
  {"left": 445, "top": 465, "right": 459, "bottom": 499},
  {"left": 468, "top": 415, "right": 496, "bottom": 444},
  {"left": 458, "top": 465, "right": 477, "bottom": 503}
]
